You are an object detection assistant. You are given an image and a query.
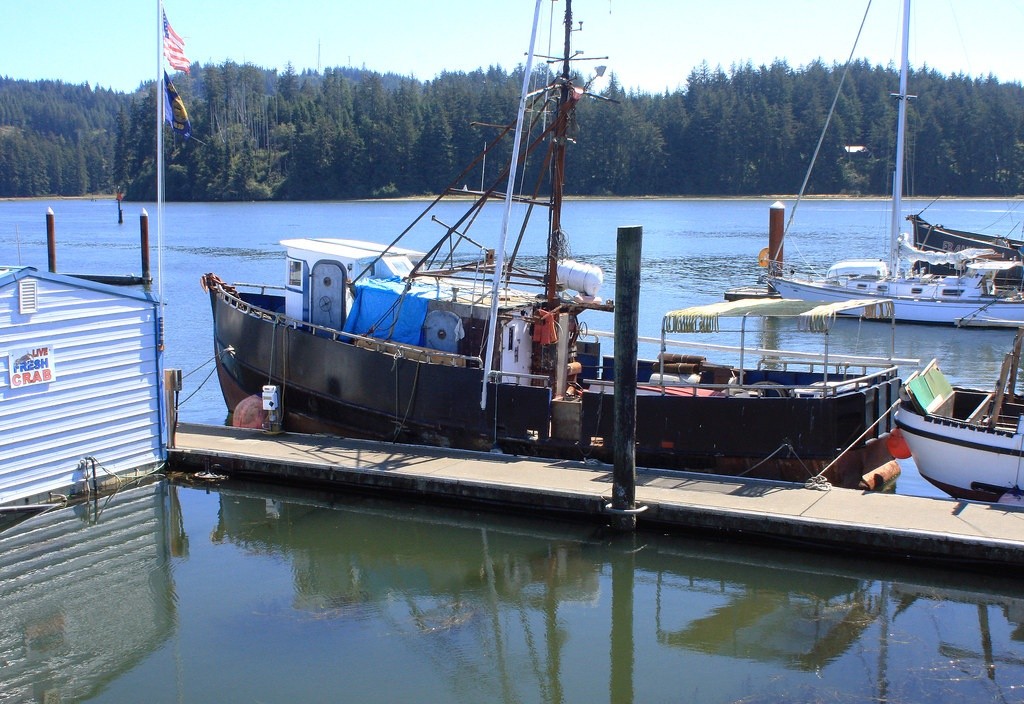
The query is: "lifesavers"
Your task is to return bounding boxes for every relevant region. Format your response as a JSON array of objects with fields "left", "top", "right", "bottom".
[{"left": 758, "top": 247, "right": 769, "bottom": 266}]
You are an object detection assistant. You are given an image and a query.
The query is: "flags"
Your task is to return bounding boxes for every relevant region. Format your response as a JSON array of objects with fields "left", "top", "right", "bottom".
[
  {"left": 161, "top": 10, "right": 191, "bottom": 73},
  {"left": 163, "top": 69, "right": 192, "bottom": 144}
]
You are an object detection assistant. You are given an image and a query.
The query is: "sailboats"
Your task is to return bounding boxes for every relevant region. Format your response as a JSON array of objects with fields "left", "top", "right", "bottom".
[
  {"left": 199, "top": 0, "right": 922, "bottom": 495},
  {"left": 749, "top": 0, "right": 1024, "bottom": 331}
]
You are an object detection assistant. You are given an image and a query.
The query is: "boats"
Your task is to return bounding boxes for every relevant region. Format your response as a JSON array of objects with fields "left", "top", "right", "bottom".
[
  {"left": 903, "top": 122, "right": 1024, "bottom": 294},
  {"left": 892, "top": 286, "right": 1024, "bottom": 508}
]
